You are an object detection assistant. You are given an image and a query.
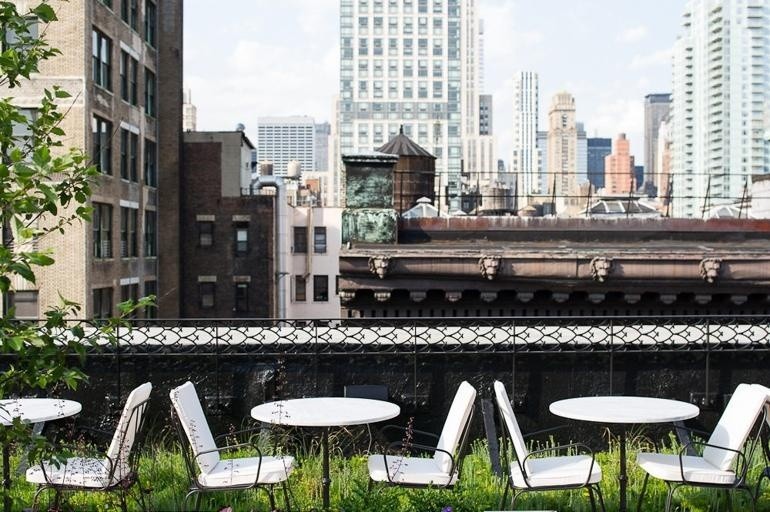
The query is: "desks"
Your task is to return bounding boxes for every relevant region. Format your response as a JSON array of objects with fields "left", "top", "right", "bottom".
[
  {"left": 251, "top": 398, "right": 400, "bottom": 509},
  {"left": 549, "top": 396, "right": 700, "bottom": 512},
  {"left": 0, "top": 398, "right": 82, "bottom": 512}
]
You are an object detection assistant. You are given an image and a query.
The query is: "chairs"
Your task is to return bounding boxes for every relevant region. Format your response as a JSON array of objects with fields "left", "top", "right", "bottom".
[
  {"left": 26, "top": 382, "right": 152, "bottom": 512},
  {"left": 367, "top": 381, "right": 477, "bottom": 493},
  {"left": 494, "top": 381, "right": 606, "bottom": 512},
  {"left": 750, "top": 384, "right": 770, "bottom": 500},
  {"left": 637, "top": 384, "right": 768, "bottom": 512},
  {"left": 169, "top": 381, "right": 295, "bottom": 512}
]
